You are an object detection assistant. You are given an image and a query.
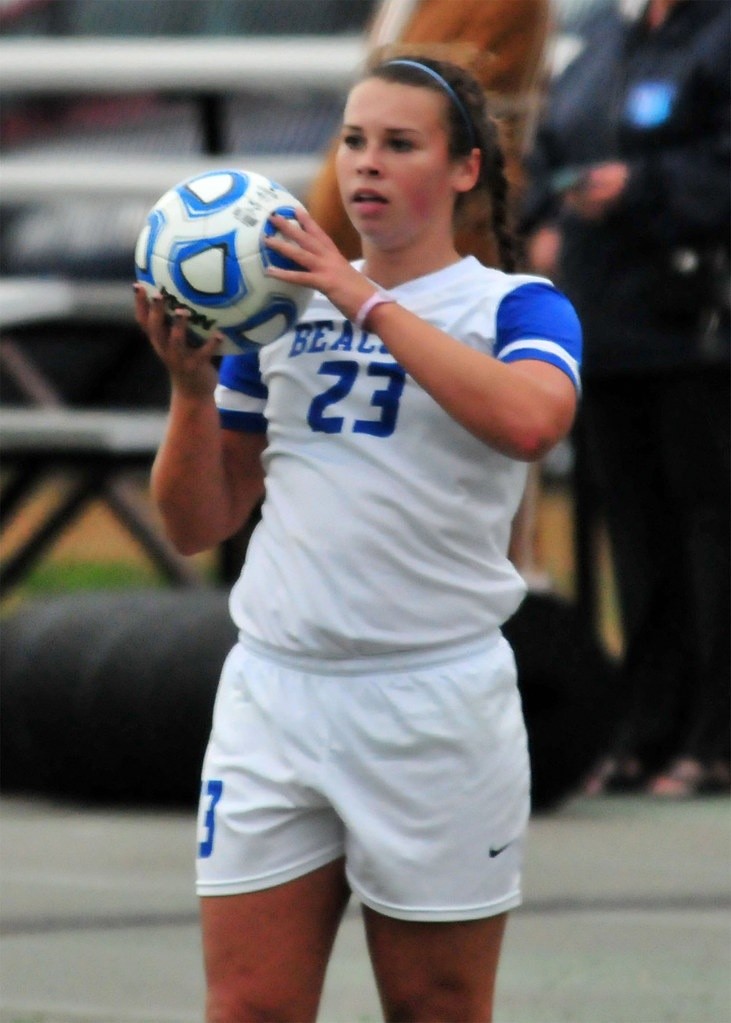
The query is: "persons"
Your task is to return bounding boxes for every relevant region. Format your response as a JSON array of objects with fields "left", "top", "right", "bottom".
[
  {"left": 131, "top": 55, "right": 587, "bottom": 1023},
  {"left": 301, "top": 1, "right": 731, "bottom": 805}
]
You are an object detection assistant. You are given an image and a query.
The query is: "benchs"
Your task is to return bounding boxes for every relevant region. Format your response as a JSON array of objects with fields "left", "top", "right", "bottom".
[{"left": 0, "top": 38, "right": 590, "bottom": 608}]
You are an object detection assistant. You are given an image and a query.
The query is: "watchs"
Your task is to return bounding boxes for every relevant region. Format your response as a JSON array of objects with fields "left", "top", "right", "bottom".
[{"left": 355, "top": 294, "right": 398, "bottom": 330}]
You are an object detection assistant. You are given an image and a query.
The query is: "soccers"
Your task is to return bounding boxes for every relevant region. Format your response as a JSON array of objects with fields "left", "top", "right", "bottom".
[{"left": 135, "top": 166, "right": 315, "bottom": 360}]
